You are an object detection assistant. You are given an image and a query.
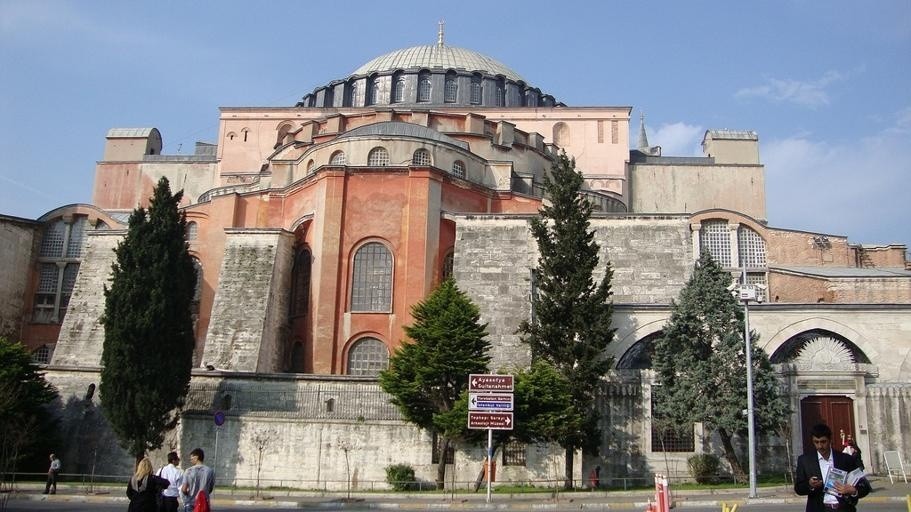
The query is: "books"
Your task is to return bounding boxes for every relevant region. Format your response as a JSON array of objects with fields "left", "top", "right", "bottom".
[{"left": 824, "top": 468, "right": 846, "bottom": 497}]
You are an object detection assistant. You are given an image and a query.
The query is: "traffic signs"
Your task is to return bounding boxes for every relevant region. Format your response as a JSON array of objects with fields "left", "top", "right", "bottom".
[
  {"left": 469, "top": 412, "right": 514, "bottom": 430},
  {"left": 469, "top": 374, "right": 514, "bottom": 391},
  {"left": 468, "top": 393, "right": 514, "bottom": 411}
]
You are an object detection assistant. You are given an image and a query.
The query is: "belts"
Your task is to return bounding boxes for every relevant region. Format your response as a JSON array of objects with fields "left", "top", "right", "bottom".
[{"left": 824, "top": 503, "right": 839, "bottom": 509}]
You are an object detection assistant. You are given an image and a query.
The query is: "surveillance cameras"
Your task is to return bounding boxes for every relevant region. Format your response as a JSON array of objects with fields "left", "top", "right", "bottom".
[
  {"left": 725, "top": 283, "right": 739, "bottom": 293},
  {"left": 755, "top": 282, "right": 767, "bottom": 290},
  {"left": 757, "top": 296, "right": 763, "bottom": 304}
]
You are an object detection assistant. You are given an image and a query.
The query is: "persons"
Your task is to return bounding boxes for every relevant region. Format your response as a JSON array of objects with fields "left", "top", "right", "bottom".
[
  {"left": 155, "top": 451, "right": 183, "bottom": 512},
  {"left": 590, "top": 465, "right": 601, "bottom": 488},
  {"left": 42, "top": 454, "right": 62, "bottom": 494},
  {"left": 181, "top": 449, "right": 216, "bottom": 512},
  {"left": 841, "top": 444, "right": 865, "bottom": 471},
  {"left": 126, "top": 457, "right": 168, "bottom": 512},
  {"left": 843, "top": 433, "right": 856, "bottom": 447},
  {"left": 794, "top": 422, "right": 872, "bottom": 512}
]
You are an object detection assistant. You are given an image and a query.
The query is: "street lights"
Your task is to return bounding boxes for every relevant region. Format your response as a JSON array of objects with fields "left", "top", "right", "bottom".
[{"left": 728, "top": 258, "right": 770, "bottom": 499}]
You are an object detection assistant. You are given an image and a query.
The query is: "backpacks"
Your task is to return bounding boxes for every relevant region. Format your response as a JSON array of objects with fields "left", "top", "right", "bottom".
[{"left": 193, "top": 490, "right": 210, "bottom": 512}]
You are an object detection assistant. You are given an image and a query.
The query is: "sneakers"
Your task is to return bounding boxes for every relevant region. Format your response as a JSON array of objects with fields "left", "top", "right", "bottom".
[{"left": 42, "top": 490, "right": 57, "bottom": 495}]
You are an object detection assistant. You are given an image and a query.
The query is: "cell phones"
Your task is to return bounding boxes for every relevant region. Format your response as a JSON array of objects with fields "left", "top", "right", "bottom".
[{"left": 812, "top": 477, "right": 818, "bottom": 480}]
